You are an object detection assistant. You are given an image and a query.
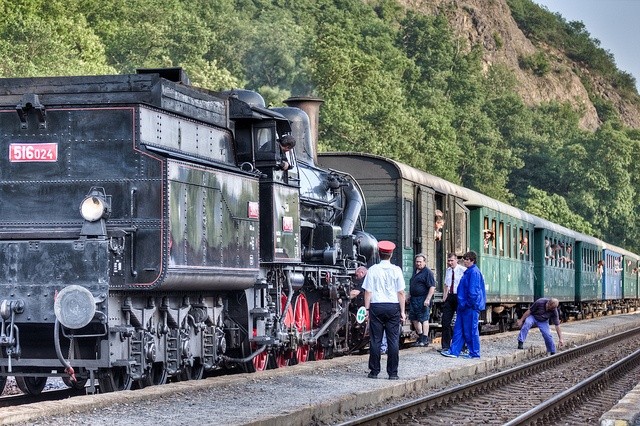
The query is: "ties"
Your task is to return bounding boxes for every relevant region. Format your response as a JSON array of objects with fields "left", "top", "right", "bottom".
[{"left": 450, "top": 269, "right": 455, "bottom": 294}]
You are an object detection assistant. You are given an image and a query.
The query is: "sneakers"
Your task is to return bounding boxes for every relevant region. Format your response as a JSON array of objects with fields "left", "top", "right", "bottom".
[
  {"left": 410, "top": 341, "right": 420, "bottom": 347},
  {"left": 462, "top": 354, "right": 480, "bottom": 360},
  {"left": 416, "top": 341, "right": 429, "bottom": 347},
  {"left": 551, "top": 352, "right": 555, "bottom": 355},
  {"left": 389, "top": 376, "right": 400, "bottom": 380},
  {"left": 518, "top": 341, "right": 523, "bottom": 349},
  {"left": 368, "top": 372, "right": 378, "bottom": 378},
  {"left": 440, "top": 351, "right": 457, "bottom": 357}
]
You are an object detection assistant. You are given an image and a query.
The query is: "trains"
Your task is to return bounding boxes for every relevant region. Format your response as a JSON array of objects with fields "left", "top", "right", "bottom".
[{"left": 0, "top": 66, "right": 640, "bottom": 394}]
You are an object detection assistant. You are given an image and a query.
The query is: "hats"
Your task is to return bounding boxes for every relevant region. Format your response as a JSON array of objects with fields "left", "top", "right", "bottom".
[{"left": 377, "top": 240, "right": 396, "bottom": 252}]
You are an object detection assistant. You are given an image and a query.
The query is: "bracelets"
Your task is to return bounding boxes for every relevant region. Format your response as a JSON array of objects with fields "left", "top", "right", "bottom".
[{"left": 366, "top": 309, "right": 370, "bottom": 310}]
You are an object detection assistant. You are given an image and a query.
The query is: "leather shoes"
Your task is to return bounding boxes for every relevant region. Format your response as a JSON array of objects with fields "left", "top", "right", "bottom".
[
  {"left": 462, "top": 348, "right": 465, "bottom": 351},
  {"left": 437, "top": 349, "right": 448, "bottom": 351}
]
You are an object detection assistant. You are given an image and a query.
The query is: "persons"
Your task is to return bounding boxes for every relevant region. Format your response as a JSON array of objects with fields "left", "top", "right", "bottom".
[
  {"left": 512, "top": 297, "right": 565, "bottom": 357},
  {"left": 435, "top": 210, "right": 445, "bottom": 241},
  {"left": 437, "top": 252, "right": 469, "bottom": 352},
  {"left": 483, "top": 230, "right": 495, "bottom": 249},
  {"left": 549, "top": 238, "right": 574, "bottom": 264},
  {"left": 440, "top": 251, "right": 486, "bottom": 359},
  {"left": 520, "top": 237, "right": 528, "bottom": 255},
  {"left": 545, "top": 240, "right": 550, "bottom": 259},
  {"left": 405, "top": 254, "right": 436, "bottom": 347},
  {"left": 598, "top": 260, "right": 604, "bottom": 279},
  {"left": 361, "top": 241, "right": 407, "bottom": 380},
  {"left": 350, "top": 266, "right": 368, "bottom": 354}
]
